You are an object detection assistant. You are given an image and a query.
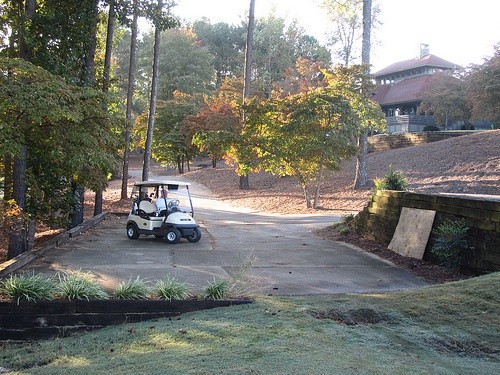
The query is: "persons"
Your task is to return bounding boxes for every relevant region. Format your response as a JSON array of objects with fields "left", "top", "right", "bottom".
[{"left": 155, "top": 190, "right": 179, "bottom": 218}]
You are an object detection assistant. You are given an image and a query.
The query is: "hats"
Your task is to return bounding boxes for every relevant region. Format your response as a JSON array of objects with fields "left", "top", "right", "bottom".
[{"left": 160, "top": 189, "right": 170, "bottom": 194}]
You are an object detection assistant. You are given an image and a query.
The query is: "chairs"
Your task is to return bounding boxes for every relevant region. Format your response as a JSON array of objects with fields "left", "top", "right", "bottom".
[{"left": 138, "top": 200, "right": 165, "bottom": 221}]
